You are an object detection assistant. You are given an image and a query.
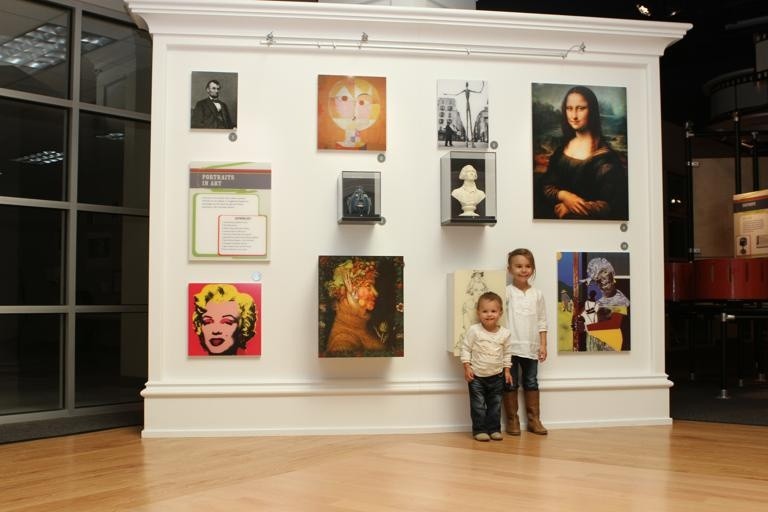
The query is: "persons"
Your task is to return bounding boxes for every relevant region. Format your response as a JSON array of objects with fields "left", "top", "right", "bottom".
[
  {"left": 451, "top": 165, "right": 488, "bottom": 217},
  {"left": 191, "top": 80, "right": 238, "bottom": 129},
  {"left": 533, "top": 86, "right": 628, "bottom": 219},
  {"left": 443, "top": 81, "right": 487, "bottom": 148},
  {"left": 461, "top": 293, "right": 513, "bottom": 442},
  {"left": 461, "top": 272, "right": 505, "bottom": 361},
  {"left": 498, "top": 248, "right": 551, "bottom": 437},
  {"left": 321, "top": 258, "right": 397, "bottom": 355},
  {"left": 192, "top": 284, "right": 257, "bottom": 355}
]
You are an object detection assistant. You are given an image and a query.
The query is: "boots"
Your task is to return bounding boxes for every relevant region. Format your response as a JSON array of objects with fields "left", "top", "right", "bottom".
[
  {"left": 524, "top": 389, "right": 547, "bottom": 435},
  {"left": 504, "top": 391, "right": 521, "bottom": 435}
]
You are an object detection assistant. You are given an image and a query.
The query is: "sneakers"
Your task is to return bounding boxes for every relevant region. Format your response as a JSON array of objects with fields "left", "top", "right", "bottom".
[
  {"left": 490, "top": 432, "right": 503, "bottom": 440},
  {"left": 473, "top": 432, "right": 490, "bottom": 441}
]
